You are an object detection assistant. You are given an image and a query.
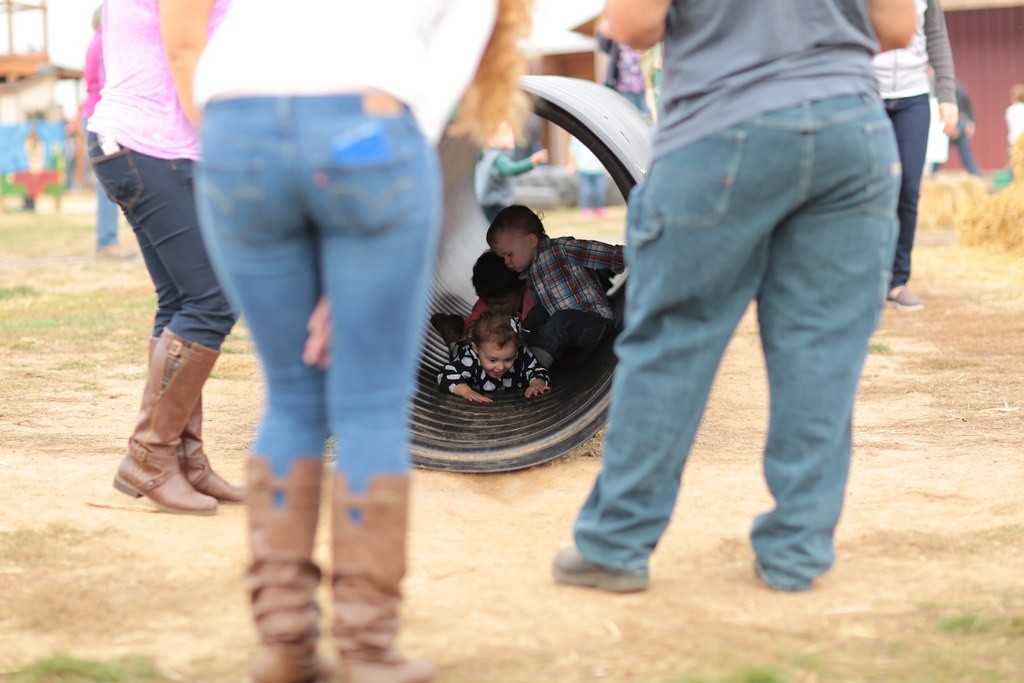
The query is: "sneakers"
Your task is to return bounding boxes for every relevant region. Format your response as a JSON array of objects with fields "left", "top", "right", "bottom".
[
  {"left": 551, "top": 543, "right": 647, "bottom": 592},
  {"left": 887, "top": 287, "right": 925, "bottom": 313}
]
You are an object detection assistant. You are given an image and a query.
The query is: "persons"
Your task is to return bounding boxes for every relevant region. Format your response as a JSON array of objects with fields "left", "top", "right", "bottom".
[
  {"left": 872, "top": 0, "right": 960, "bottom": 314},
  {"left": 594, "top": 19, "right": 652, "bottom": 115},
  {"left": 487, "top": 205, "right": 627, "bottom": 372},
  {"left": 933, "top": 80, "right": 981, "bottom": 179},
  {"left": 475, "top": 123, "right": 550, "bottom": 219},
  {"left": 429, "top": 313, "right": 552, "bottom": 404},
  {"left": 464, "top": 247, "right": 537, "bottom": 335},
  {"left": 994, "top": 81, "right": 1024, "bottom": 185},
  {"left": 191, "top": 0, "right": 532, "bottom": 681},
  {"left": 568, "top": 128, "right": 609, "bottom": 219},
  {"left": 77, "top": 4, "right": 135, "bottom": 260},
  {"left": 552, "top": 0, "right": 919, "bottom": 592},
  {"left": 86, "top": 0, "right": 248, "bottom": 514}
]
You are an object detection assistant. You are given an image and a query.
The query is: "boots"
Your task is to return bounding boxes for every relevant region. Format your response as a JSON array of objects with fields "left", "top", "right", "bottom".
[
  {"left": 113, "top": 329, "right": 218, "bottom": 512},
  {"left": 149, "top": 334, "right": 245, "bottom": 502},
  {"left": 333, "top": 469, "right": 434, "bottom": 683},
  {"left": 244, "top": 453, "right": 333, "bottom": 683}
]
